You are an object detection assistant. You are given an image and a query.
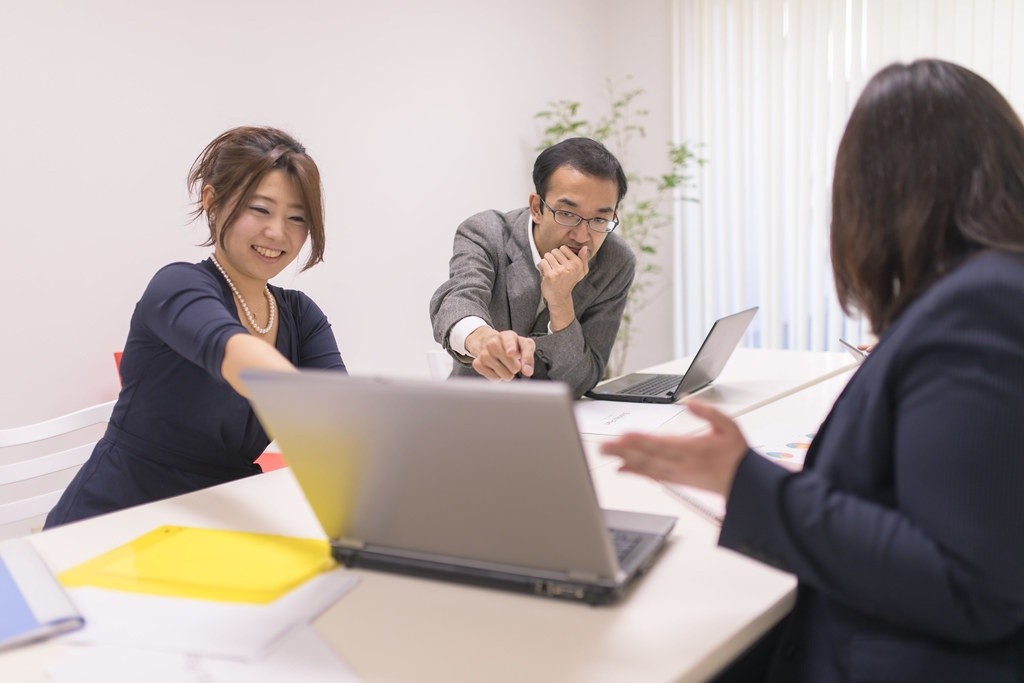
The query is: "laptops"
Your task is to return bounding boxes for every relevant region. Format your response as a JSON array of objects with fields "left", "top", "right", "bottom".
[
  {"left": 237, "top": 367, "right": 679, "bottom": 608},
  {"left": 838, "top": 338, "right": 868, "bottom": 364},
  {"left": 582, "top": 306, "right": 760, "bottom": 404}
]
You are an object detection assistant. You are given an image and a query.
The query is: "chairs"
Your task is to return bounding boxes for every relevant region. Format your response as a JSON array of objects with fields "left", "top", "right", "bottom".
[{"left": 0, "top": 394, "right": 122, "bottom": 524}]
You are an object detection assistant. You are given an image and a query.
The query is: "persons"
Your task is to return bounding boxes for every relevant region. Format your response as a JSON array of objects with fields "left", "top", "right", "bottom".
[
  {"left": 601, "top": 58, "right": 1023, "bottom": 683},
  {"left": 430, "top": 137, "right": 637, "bottom": 400},
  {"left": 43, "top": 126, "right": 350, "bottom": 530}
]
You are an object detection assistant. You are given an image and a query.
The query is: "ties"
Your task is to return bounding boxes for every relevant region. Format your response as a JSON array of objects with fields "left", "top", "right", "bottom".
[{"left": 533, "top": 298, "right": 550, "bottom": 335}]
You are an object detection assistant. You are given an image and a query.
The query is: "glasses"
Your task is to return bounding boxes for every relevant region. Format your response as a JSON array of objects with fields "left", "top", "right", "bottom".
[{"left": 539, "top": 194, "right": 620, "bottom": 233}]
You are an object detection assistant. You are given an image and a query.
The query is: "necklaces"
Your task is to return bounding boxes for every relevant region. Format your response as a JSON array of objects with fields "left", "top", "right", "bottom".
[{"left": 210, "top": 252, "right": 275, "bottom": 334}]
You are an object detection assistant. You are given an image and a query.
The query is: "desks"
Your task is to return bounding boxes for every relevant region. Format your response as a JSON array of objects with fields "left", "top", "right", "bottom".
[{"left": 0, "top": 349, "right": 874, "bottom": 683}]
